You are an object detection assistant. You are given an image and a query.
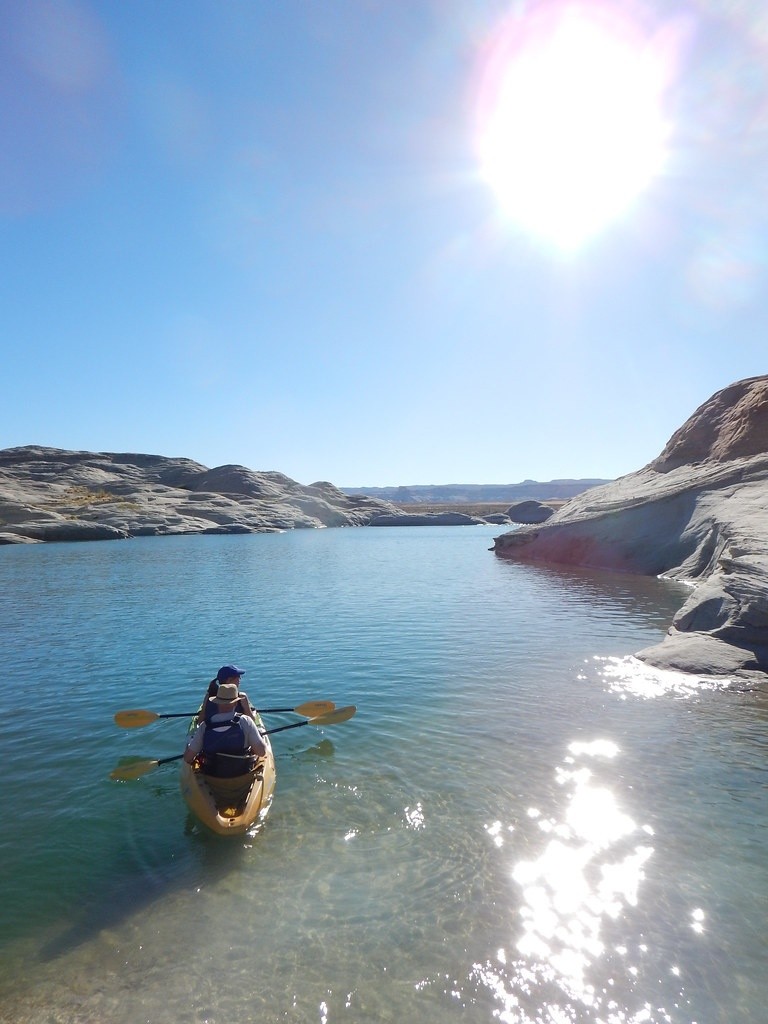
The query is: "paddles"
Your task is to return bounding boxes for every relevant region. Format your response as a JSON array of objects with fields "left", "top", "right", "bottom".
[
  {"left": 108, "top": 704, "right": 358, "bottom": 781},
  {"left": 113, "top": 699, "right": 337, "bottom": 729}
]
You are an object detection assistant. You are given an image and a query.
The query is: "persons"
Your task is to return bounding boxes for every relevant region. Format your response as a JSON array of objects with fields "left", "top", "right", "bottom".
[
  {"left": 195, "top": 665, "right": 257, "bottom": 725},
  {"left": 184, "top": 683, "right": 265, "bottom": 804}
]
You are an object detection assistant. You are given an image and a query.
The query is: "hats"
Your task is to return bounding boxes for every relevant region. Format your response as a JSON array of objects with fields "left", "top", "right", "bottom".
[
  {"left": 209, "top": 684, "right": 247, "bottom": 704},
  {"left": 217, "top": 665, "right": 245, "bottom": 678}
]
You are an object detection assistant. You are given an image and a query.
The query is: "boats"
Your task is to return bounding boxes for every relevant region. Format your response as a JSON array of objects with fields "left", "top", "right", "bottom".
[{"left": 180, "top": 692, "right": 277, "bottom": 839}]
